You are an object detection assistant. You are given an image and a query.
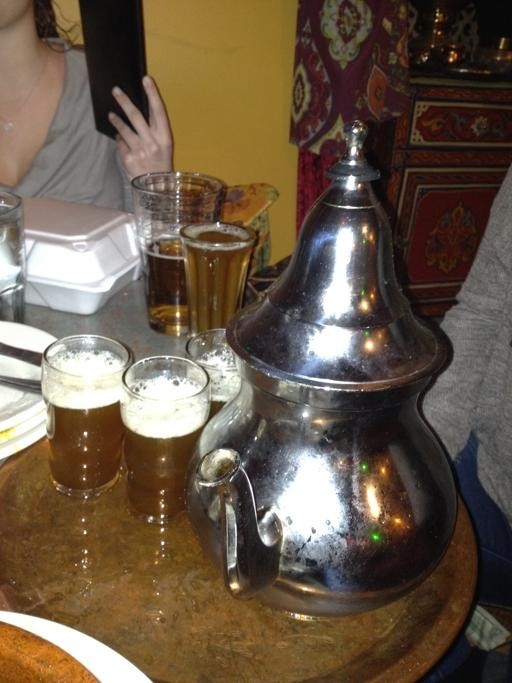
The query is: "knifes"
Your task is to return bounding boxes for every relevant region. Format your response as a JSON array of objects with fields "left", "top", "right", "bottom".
[
  {"left": 1, "top": 371, "right": 50, "bottom": 395},
  {"left": 0, "top": 340, "right": 47, "bottom": 368}
]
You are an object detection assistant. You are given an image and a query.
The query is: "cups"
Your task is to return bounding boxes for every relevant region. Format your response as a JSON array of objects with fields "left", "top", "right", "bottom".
[
  {"left": 39, "top": 334, "right": 128, "bottom": 498},
  {"left": 128, "top": 168, "right": 225, "bottom": 336},
  {"left": 187, "top": 327, "right": 241, "bottom": 419},
  {"left": 181, "top": 214, "right": 257, "bottom": 329},
  {"left": 117, "top": 350, "right": 214, "bottom": 522},
  {"left": 0, "top": 188, "right": 27, "bottom": 323}
]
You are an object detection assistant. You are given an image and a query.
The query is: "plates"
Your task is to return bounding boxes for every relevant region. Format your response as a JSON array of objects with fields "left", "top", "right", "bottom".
[{"left": 0, "top": 316, "right": 61, "bottom": 460}]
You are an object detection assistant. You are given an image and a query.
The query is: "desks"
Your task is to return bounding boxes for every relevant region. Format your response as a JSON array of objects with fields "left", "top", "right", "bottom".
[{"left": 0, "top": 274, "right": 480, "bottom": 683}]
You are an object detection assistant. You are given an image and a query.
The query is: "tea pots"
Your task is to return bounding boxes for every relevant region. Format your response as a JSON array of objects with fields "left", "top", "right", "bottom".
[{"left": 189, "top": 116, "right": 462, "bottom": 622}]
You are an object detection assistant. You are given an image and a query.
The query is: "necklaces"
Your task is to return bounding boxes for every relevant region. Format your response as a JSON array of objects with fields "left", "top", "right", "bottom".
[{"left": 0, "top": 42, "right": 56, "bottom": 144}]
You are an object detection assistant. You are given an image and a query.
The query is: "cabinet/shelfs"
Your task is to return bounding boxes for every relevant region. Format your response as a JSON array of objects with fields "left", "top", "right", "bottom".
[{"left": 292, "top": 0, "right": 512, "bottom": 318}]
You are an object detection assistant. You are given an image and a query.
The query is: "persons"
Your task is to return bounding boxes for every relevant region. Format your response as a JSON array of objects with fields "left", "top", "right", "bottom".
[
  {"left": 422, "top": 158, "right": 511, "bottom": 680},
  {"left": 1, "top": 0, "right": 177, "bottom": 242}
]
